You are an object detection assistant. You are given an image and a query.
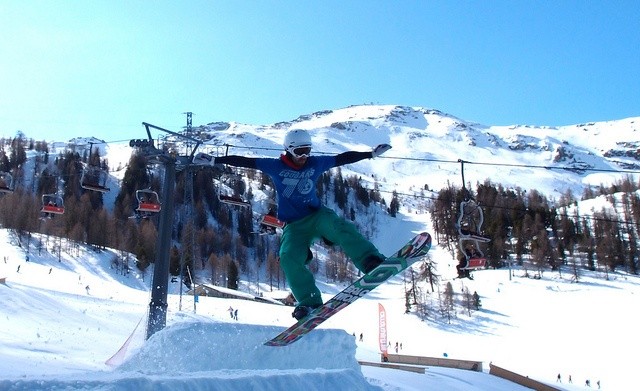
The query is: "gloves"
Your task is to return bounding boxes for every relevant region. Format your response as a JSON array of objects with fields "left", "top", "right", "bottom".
[
  {"left": 372, "top": 143, "right": 392, "bottom": 158},
  {"left": 192, "top": 152, "right": 215, "bottom": 166}
]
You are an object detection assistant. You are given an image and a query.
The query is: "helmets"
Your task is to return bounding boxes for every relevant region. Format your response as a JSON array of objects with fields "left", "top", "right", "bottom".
[{"left": 285, "top": 129, "right": 312, "bottom": 158}]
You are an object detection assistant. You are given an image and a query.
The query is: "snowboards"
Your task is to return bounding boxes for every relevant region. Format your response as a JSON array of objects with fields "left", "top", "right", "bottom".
[{"left": 263, "top": 232, "right": 432, "bottom": 347}]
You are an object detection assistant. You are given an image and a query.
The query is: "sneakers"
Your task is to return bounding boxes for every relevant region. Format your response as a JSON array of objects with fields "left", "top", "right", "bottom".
[{"left": 291, "top": 304, "right": 323, "bottom": 320}]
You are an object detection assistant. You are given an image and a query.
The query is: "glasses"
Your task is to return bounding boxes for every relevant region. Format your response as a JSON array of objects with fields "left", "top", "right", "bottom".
[{"left": 288, "top": 145, "right": 314, "bottom": 157}]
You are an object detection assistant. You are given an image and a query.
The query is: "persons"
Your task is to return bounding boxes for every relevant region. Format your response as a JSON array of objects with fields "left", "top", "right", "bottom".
[
  {"left": 360, "top": 332, "right": 364, "bottom": 342},
  {"left": 38, "top": 202, "right": 57, "bottom": 222},
  {"left": 261, "top": 209, "right": 277, "bottom": 236},
  {"left": 457, "top": 249, "right": 480, "bottom": 276},
  {"left": 234, "top": 309, "right": 238, "bottom": 320},
  {"left": 228, "top": 306, "right": 234, "bottom": 318},
  {"left": 192, "top": 131, "right": 393, "bottom": 322},
  {"left": 130, "top": 199, "right": 154, "bottom": 220}
]
